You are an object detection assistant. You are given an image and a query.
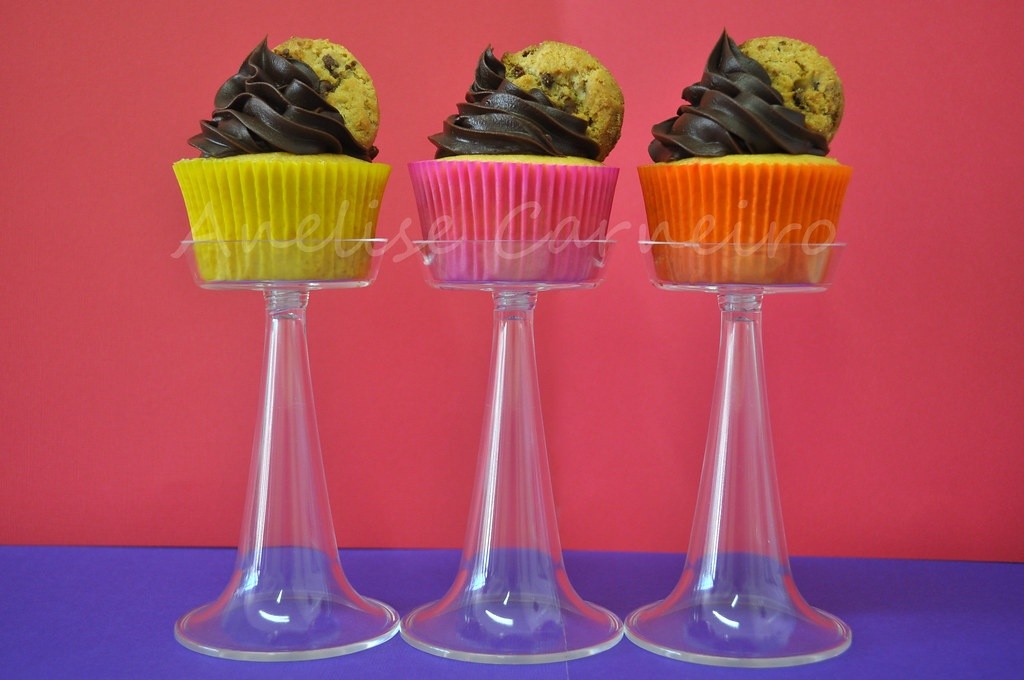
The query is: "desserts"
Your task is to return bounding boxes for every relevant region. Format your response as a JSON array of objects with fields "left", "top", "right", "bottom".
[
  {"left": 637, "top": 25, "right": 854, "bottom": 288},
  {"left": 405, "top": 41, "right": 626, "bottom": 284},
  {"left": 171, "top": 32, "right": 391, "bottom": 281}
]
